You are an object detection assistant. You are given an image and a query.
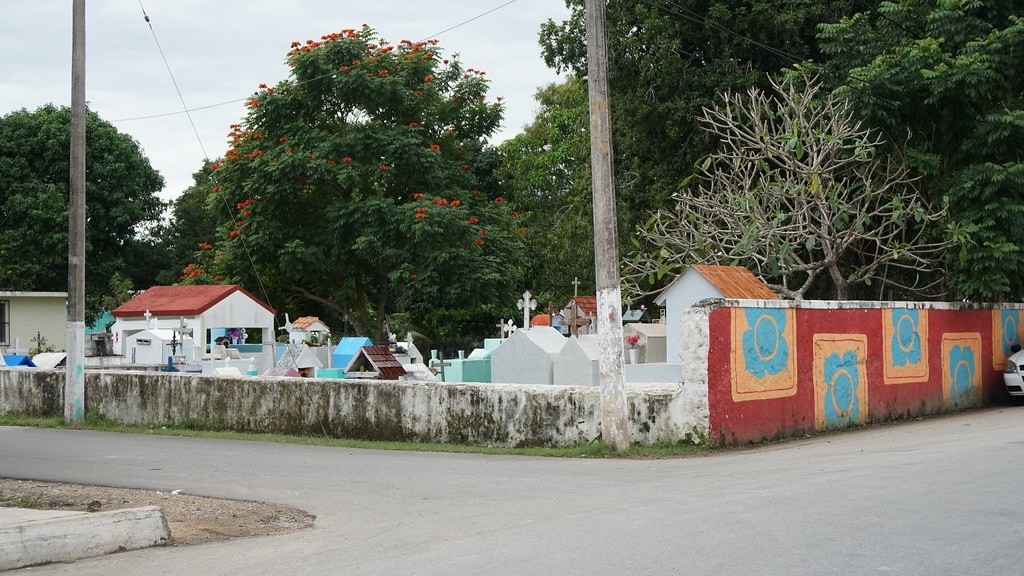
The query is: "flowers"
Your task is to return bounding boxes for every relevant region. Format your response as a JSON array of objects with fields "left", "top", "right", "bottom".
[
  {"left": 241, "top": 328, "right": 248, "bottom": 338},
  {"left": 228, "top": 328, "right": 243, "bottom": 340},
  {"left": 626, "top": 334, "right": 640, "bottom": 349}
]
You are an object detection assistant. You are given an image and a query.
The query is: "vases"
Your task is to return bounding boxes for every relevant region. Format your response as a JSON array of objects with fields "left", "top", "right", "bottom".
[
  {"left": 239, "top": 339, "right": 246, "bottom": 344},
  {"left": 232, "top": 337, "right": 238, "bottom": 344},
  {"left": 629, "top": 349, "right": 640, "bottom": 364}
]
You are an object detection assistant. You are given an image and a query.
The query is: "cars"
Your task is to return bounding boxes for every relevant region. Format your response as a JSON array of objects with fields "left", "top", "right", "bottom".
[{"left": 1003, "top": 345, "right": 1023, "bottom": 397}]
